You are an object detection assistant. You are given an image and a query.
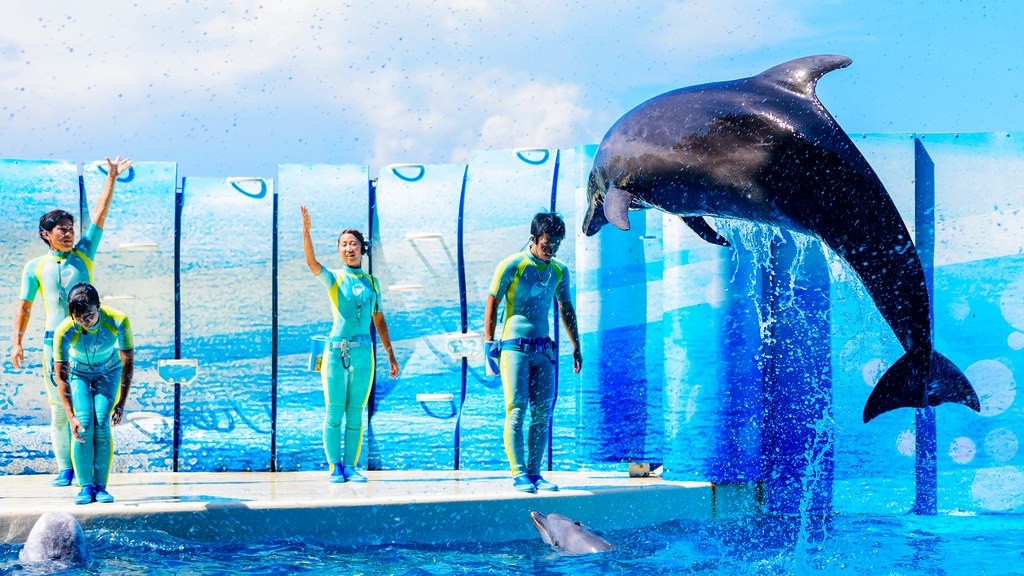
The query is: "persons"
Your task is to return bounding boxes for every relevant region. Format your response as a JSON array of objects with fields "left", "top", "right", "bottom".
[
  {"left": 301, "top": 204, "right": 398, "bottom": 484},
  {"left": 10, "top": 153, "right": 135, "bottom": 487},
  {"left": 51, "top": 284, "right": 136, "bottom": 504},
  {"left": 483, "top": 212, "right": 584, "bottom": 494}
]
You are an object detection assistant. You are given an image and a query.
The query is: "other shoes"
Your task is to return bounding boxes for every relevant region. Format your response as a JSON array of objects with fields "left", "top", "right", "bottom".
[
  {"left": 53, "top": 469, "right": 73, "bottom": 486},
  {"left": 74, "top": 485, "right": 93, "bottom": 505},
  {"left": 532, "top": 475, "right": 559, "bottom": 489},
  {"left": 328, "top": 464, "right": 345, "bottom": 483},
  {"left": 343, "top": 463, "right": 368, "bottom": 482},
  {"left": 513, "top": 476, "right": 537, "bottom": 493},
  {"left": 95, "top": 488, "right": 114, "bottom": 504}
]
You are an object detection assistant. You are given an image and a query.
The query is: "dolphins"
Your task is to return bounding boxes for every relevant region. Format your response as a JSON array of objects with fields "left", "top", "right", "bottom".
[
  {"left": 19, "top": 510, "right": 88, "bottom": 568},
  {"left": 531, "top": 510, "right": 615, "bottom": 557},
  {"left": 580, "top": 52, "right": 984, "bottom": 424}
]
